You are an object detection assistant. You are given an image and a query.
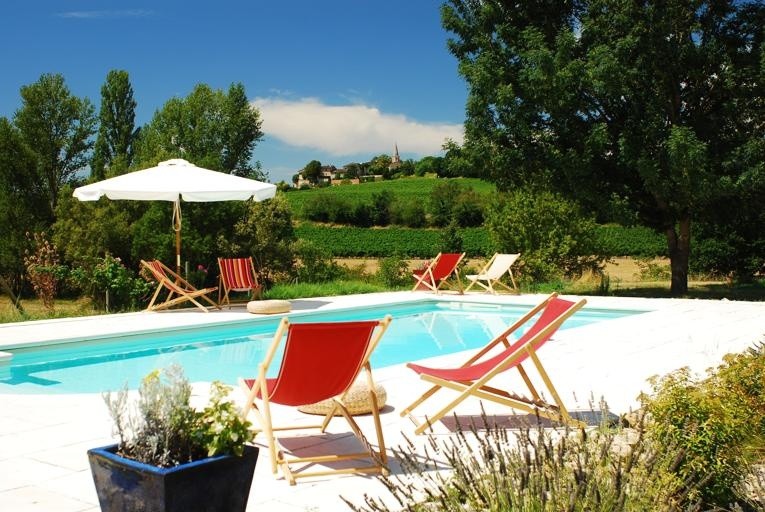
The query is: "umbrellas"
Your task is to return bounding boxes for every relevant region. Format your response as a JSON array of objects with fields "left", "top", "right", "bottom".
[{"left": 68, "top": 158, "right": 278, "bottom": 288}]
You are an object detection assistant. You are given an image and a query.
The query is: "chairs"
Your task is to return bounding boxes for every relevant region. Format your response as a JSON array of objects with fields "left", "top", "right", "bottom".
[
  {"left": 399, "top": 292, "right": 588, "bottom": 433},
  {"left": 140, "top": 254, "right": 265, "bottom": 315},
  {"left": 412, "top": 250, "right": 524, "bottom": 295},
  {"left": 239, "top": 314, "right": 394, "bottom": 484}
]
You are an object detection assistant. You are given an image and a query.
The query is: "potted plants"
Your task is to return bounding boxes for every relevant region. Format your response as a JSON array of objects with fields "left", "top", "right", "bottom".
[{"left": 86, "top": 363, "right": 260, "bottom": 512}]
